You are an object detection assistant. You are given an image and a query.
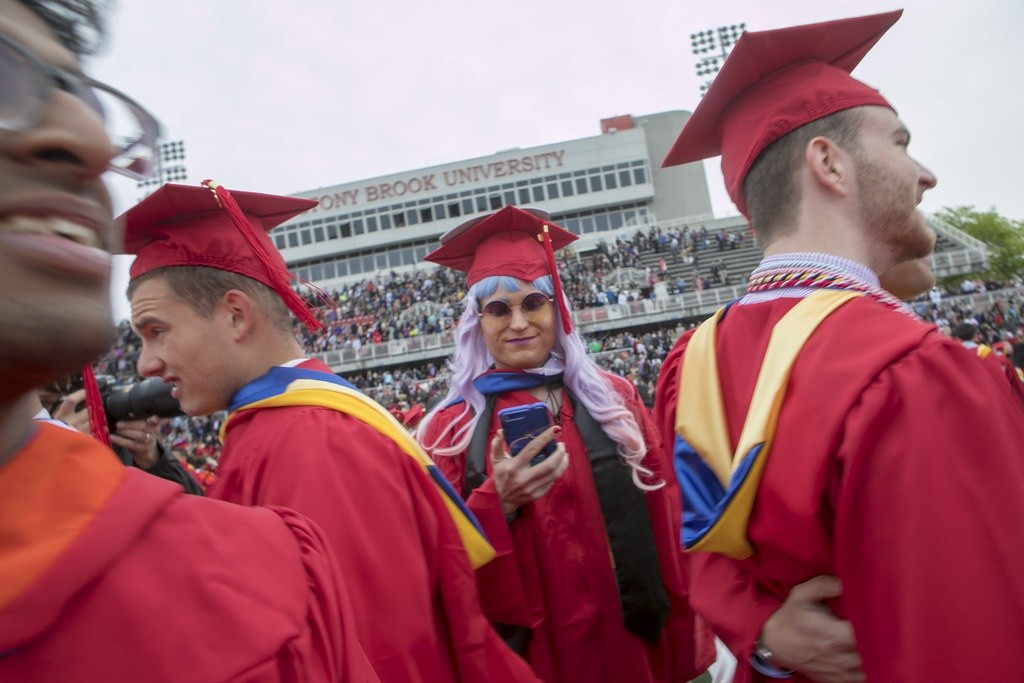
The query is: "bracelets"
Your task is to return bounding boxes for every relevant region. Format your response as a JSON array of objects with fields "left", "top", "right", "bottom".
[{"left": 752, "top": 625, "right": 790, "bottom": 672}]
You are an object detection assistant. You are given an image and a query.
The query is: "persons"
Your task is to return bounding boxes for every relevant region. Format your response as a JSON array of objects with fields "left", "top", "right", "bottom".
[
  {"left": 414, "top": 205, "right": 719, "bottom": 683},
  {"left": 39, "top": 222, "right": 1024, "bottom": 497},
  {"left": 652, "top": 8, "right": 1024, "bottom": 683},
  {"left": 109, "top": 180, "right": 539, "bottom": 683},
  {"left": 0, "top": 1, "right": 382, "bottom": 683}
]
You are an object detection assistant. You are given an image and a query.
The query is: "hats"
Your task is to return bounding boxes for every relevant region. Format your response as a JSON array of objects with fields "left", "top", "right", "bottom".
[
  {"left": 109, "top": 178, "right": 337, "bottom": 335},
  {"left": 660, "top": 8, "right": 904, "bottom": 237},
  {"left": 422, "top": 204, "right": 580, "bottom": 335}
]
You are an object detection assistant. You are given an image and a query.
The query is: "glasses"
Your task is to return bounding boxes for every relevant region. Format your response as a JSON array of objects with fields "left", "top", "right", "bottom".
[
  {"left": 476, "top": 291, "right": 555, "bottom": 330},
  {"left": 0, "top": 33, "right": 164, "bottom": 181}
]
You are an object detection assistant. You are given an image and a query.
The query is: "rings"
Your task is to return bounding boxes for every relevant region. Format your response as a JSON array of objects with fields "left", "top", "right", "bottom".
[{"left": 144, "top": 432, "right": 150, "bottom": 444}]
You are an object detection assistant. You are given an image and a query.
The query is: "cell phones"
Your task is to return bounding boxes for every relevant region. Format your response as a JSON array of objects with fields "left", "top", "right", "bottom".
[{"left": 497, "top": 401, "right": 557, "bottom": 467}]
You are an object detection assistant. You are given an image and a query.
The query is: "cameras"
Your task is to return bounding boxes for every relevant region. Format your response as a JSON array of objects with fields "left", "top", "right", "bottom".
[{"left": 70, "top": 372, "right": 187, "bottom": 437}]
